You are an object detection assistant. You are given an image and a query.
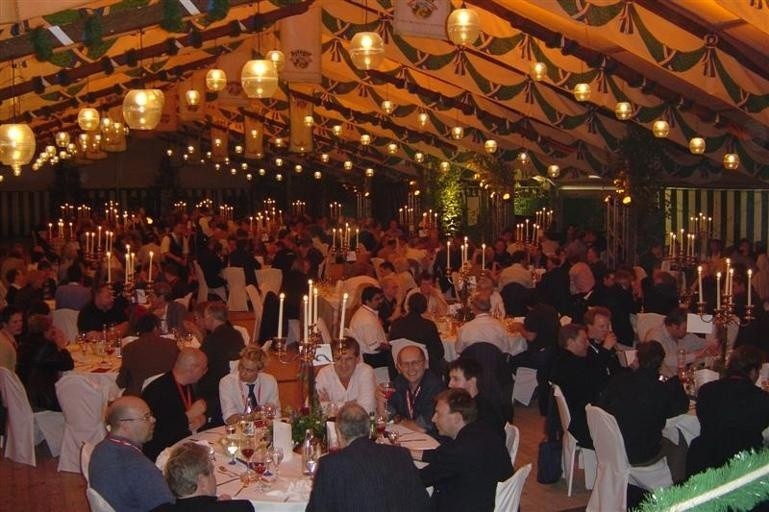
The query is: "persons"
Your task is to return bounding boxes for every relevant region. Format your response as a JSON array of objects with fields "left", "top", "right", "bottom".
[
  {"left": 191, "top": 304, "right": 246, "bottom": 424},
  {"left": 141, "top": 282, "right": 187, "bottom": 338},
  {"left": 347, "top": 286, "right": 394, "bottom": 367},
  {"left": 644, "top": 312, "right": 709, "bottom": 381},
  {"left": 18, "top": 314, "right": 74, "bottom": 412},
  {"left": 89, "top": 396, "right": 174, "bottom": 506},
  {"left": 116, "top": 314, "right": 181, "bottom": 397},
  {"left": 2, "top": 305, "right": 22, "bottom": 374},
  {"left": 604, "top": 339, "right": 688, "bottom": 487},
  {"left": 580, "top": 306, "right": 626, "bottom": 375},
  {"left": 2, "top": 209, "right": 766, "bottom": 321},
  {"left": 555, "top": 323, "right": 619, "bottom": 451},
  {"left": 75, "top": 287, "right": 127, "bottom": 332},
  {"left": 155, "top": 442, "right": 253, "bottom": 509},
  {"left": 306, "top": 402, "right": 431, "bottom": 508},
  {"left": 220, "top": 346, "right": 281, "bottom": 425},
  {"left": 375, "top": 345, "right": 449, "bottom": 436},
  {"left": 418, "top": 387, "right": 515, "bottom": 509},
  {"left": 457, "top": 292, "right": 513, "bottom": 360},
  {"left": 304, "top": 337, "right": 376, "bottom": 423},
  {"left": 509, "top": 289, "right": 559, "bottom": 378},
  {"left": 697, "top": 346, "right": 766, "bottom": 468},
  {"left": 141, "top": 347, "right": 217, "bottom": 463},
  {"left": 386, "top": 294, "right": 443, "bottom": 377},
  {"left": 396, "top": 359, "right": 506, "bottom": 449}
]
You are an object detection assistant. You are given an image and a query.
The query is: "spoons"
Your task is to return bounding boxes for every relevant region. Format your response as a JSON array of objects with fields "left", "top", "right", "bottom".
[{"left": 234, "top": 480, "right": 249, "bottom": 496}]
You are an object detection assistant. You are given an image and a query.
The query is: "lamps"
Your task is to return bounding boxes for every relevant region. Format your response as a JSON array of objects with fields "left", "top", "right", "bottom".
[
  {"left": 185, "top": 67, "right": 199, "bottom": 107},
  {"left": 412, "top": 112, "right": 562, "bottom": 181},
  {"left": 348, "top": 10, "right": 384, "bottom": 69},
  {"left": 574, "top": 47, "right": 592, "bottom": 102},
  {"left": 615, "top": 65, "right": 632, "bottom": 120},
  {"left": 0, "top": 57, "right": 36, "bottom": 165},
  {"left": 242, "top": 11, "right": 277, "bottom": 98},
  {"left": 688, "top": 103, "right": 705, "bottom": 155},
  {"left": 166, "top": 100, "right": 397, "bottom": 184},
  {"left": 447, "top": 10, "right": 480, "bottom": 47},
  {"left": 78, "top": 74, "right": 100, "bottom": 131},
  {"left": 266, "top": 11, "right": 285, "bottom": 74},
  {"left": 204, "top": 12, "right": 226, "bottom": 92},
  {"left": 723, "top": 119, "right": 739, "bottom": 170},
  {"left": 652, "top": 83, "right": 669, "bottom": 137},
  {"left": 122, "top": 27, "right": 163, "bottom": 131},
  {"left": 13, "top": 116, "right": 130, "bottom": 178},
  {"left": 529, "top": 32, "right": 547, "bottom": 82}
]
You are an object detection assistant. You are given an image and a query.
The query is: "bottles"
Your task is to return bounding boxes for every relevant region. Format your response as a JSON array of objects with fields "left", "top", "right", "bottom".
[
  {"left": 451, "top": 318, "right": 456, "bottom": 334},
  {"left": 494, "top": 303, "right": 502, "bottom": 321},
  {"left": 115, "top": 335, "right": 123, "bottom": 358},
  {"left": 302, "top": 428, "right": 316, "bottom": 476},
  {"left": 369, "top": 412, "right": 377, "bottom": 435}
]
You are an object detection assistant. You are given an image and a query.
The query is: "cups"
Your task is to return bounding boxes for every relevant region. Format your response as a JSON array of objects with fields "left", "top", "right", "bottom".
[
  {"left": 388, "top": 431, "right": 399, "bottom": 444},
  {"left": 678, "top": 367, "right": 696, "bottom": 384},
  {"left": 171, "top": 327, "right": 194, "bottom": 345}
]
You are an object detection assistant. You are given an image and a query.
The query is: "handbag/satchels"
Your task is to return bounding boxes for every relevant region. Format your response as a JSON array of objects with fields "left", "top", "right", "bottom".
[{"left": 538, "top": 439, "right": 562, "bottom": 484}]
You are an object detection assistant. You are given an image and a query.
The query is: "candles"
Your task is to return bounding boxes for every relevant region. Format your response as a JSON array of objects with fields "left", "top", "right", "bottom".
[
  {"left": 688, "top": 207, "right": 717, "bottom": 231},
  {"left": 39, "top": 187, "right": 555, "bottom": 235}
]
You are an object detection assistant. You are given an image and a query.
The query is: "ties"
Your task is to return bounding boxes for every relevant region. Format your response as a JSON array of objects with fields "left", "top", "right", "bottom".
[{"left": 246, "top": 384, "right": 257, "bottom": 409}]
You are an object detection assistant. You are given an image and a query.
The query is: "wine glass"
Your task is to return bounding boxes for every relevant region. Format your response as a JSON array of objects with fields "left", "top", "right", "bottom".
[
  {"left": 76, "top": 322, "right": 116, "bottom": 363},
  {"left": 306, "top": 455, "right": 317, "bottom": 480},
  {"left": 379, "top": 381, "right": 395, "bottom": 414},
  {"left": 373, "top": 418, "right": 388, "bottom": 444},
  {"left": 219, "top": 404, "right": 284, "bottom": 492}
]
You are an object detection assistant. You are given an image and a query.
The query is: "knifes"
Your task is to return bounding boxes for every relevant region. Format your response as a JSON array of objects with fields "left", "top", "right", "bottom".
[
  {"left": 189, "top": 438, "right": 214, "bottom": 444},
  {"left": 216, "top": 477, "right": 239, "bottom": 487},
  {"left": 397, "top": 439, "right": 427, "bottom": 442}
]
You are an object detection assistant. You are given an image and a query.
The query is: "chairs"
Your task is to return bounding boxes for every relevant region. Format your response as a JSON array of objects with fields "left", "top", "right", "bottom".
[{"left": 3, "top": 219, "right": 768, "bottom": 511}]
